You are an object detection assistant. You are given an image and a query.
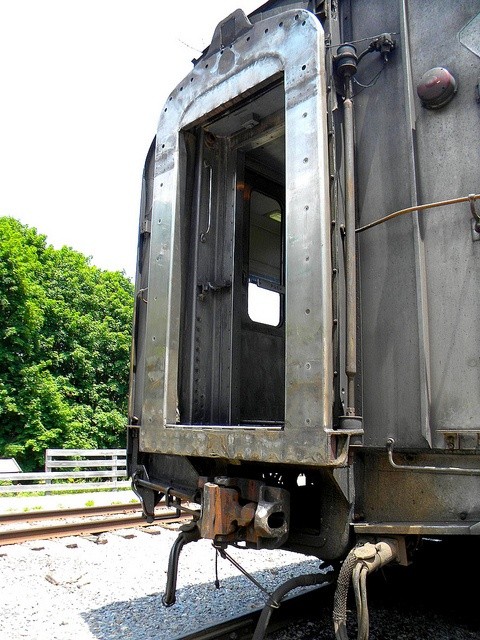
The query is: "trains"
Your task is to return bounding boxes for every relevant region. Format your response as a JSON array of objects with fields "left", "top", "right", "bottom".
[{"left": 123, "top": 0, "right": 479, "bottom": 639}]
